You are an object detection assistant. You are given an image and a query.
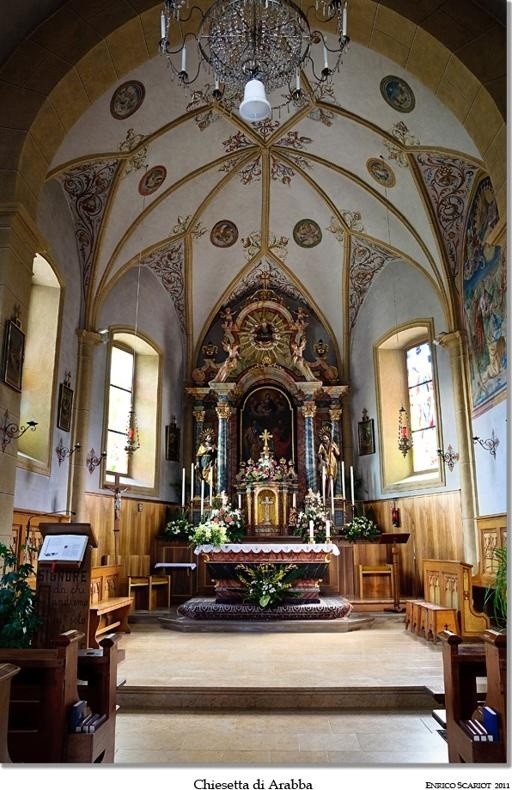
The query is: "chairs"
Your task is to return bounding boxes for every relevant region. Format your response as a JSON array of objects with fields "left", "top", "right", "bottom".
[{"left": 127, "top": 554, "right": 171, "bottom": 611}]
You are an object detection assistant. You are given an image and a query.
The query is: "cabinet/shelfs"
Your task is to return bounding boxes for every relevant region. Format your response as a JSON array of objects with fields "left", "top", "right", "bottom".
[
  {"left": 351, "top": 542, "right": 393, "bottom": 599},
  {"left": 154, "top": 539, "right": 195, "bottom": 599}
]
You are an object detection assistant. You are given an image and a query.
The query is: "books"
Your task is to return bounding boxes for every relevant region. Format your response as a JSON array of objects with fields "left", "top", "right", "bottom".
[
  {"left": 459, "top": 705, "right": 500, "bottom": 742},
  {"left": 68, "top": 700, "right": 108, "bottom": 732}
]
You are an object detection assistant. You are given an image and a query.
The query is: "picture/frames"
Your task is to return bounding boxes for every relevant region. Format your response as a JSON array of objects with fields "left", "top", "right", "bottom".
[
  {"left": 1, "top": 321, "right": 26, "bottom": 393},
  {"left": 357, "top": 419, "right": 375, "bottom": 456},
  {"left": 165, "top": 425, "right": 181, "bottom": 462},
  {"left": 57, "top": 383, "right": 74, "bottom": 432}
]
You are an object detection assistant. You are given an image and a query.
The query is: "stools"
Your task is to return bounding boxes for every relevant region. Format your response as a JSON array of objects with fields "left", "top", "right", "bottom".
[{"left": 405, "top": 600, "right": 461, "bottom": 644}]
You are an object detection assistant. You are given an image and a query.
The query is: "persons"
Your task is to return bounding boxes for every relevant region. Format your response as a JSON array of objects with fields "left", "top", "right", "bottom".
[
  {"left": 318, "top": 431, "right": 340, "bottom": 500},
  {"left": 196, "top": 432, "right": 216, "bottom": 489},
  {"left": 200, "top": 307, "right": 336, "bottom": 382}
]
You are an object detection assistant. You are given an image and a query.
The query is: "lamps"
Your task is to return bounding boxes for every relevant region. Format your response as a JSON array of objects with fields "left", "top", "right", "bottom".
[
  {"left": 124, "top": 167, "right": 147, "bottom": 458},
  {"left": 1, "top": 409, "right": 38, "bottom": 452},
  {"left": 87, "top": 448, "right": 107, "bottom": 473},
  {"left": 159, "top": 1, "right": 349, "bottom": 123},
  {"left": 55, "top": 437, "right": 82, "bottom": 464},
  {"left": 380, "top": 154, "right": 498, "bottom": 473}
]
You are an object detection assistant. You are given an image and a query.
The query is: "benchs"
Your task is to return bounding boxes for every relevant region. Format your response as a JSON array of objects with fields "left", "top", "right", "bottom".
[
  {"left": 24, "top": 564, "right": 135, "bottom": 650},
  {"left": 423, "top": 627, "right": 507, "bottom": 763},
  {"left": 0, "top": 630, "right": 125, "bottom": 763}
]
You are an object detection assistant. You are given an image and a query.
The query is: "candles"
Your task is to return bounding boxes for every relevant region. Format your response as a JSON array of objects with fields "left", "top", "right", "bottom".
[
  {"left": 181, "top": 463, "right": 242, "bottom": 516},
  {"left": 292, "top": 461, "right": 355, "bottom": 536}
]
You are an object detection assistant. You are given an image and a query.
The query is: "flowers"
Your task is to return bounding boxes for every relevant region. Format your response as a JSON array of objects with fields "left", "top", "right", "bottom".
[
  {"left": 187, "top": 507, "right": 243, "bottom": 545},
  {"left": 234, "top": 562, "right": 305, "bottom": 609},
  {"left": 242, "top": 461, "right": 289, "bottom": 481},
  {"left": 340, "top": 516, "right": 383, "bottom": 544},
  {"left": 164, "top": 519, "right": 194, "bottom": 536}
]
